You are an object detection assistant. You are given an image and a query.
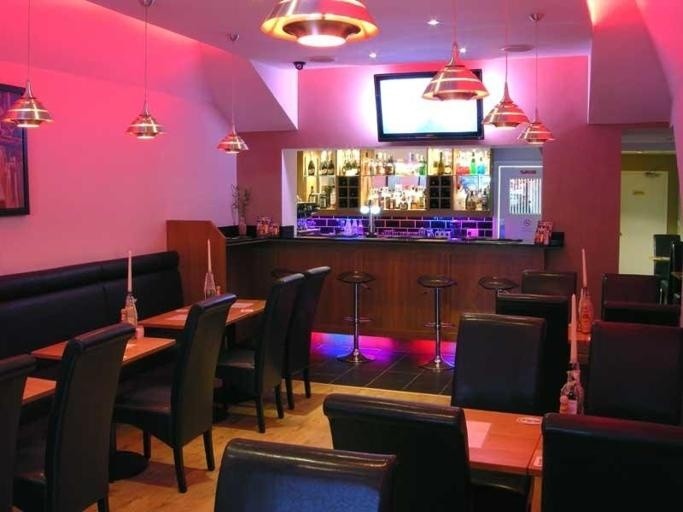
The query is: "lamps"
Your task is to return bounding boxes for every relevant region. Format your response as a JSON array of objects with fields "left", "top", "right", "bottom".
[
  {"left": 478, "top": 0, "right": 533, "bottom": 130},
  {"left": 214, "top": 26, "right": 252, "bottom": 159},
  {"left": 516, "top": 11, "right": 559, "bottom": 146},
  {"left": 254, "top": 0, "right": 381, "bottom": 54},
  {"left": 416, "top": 0, "right": 491, "bottom": 103},
  {"left": 123, "top": 1, "right": 170, "bottom": 146},
  {"left": 1, "top": 1, "right": 58, "bottom": 130}
]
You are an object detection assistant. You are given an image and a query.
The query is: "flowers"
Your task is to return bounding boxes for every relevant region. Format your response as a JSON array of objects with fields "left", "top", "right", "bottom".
[{"left": 225, "top": 181, "right": 254, "bottom": 216}]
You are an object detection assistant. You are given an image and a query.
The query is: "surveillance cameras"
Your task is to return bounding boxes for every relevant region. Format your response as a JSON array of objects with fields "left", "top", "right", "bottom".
[{"left": 293, "top": 61, "right": 305, "bottom": 70}]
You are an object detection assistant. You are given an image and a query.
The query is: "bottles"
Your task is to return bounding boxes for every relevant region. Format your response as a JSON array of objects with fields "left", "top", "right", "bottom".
[
  {"left": 419, "top": 227, "right": 425, "bottom": 237},
  {"left": 409, "top": 152, "right": 425, "bottom": 175},
  {"left": 456, "top": 185, "right": 490, "bottom": 211},
  {"left": 254, "top": 215, "right": 278, "bottom": 237},
  {"left": 203, "top": 272, "right": 215, "bottom": 299},
  {"left": 436, "top": 152, "right": 444, "bottom": 175},
  {"left": 308, "top": 184, "right": 336, "bottom": 209},
  {"left": 559, "top": 363, "right": 583, "bottom": 416},
  {"left": 365, "top": 184, "right": 425, "bottom": 211},
  {"left": 469, "top": 150, "right": 489, "bottom": 176},
  {"left": 578, "top": 287, "right": 593, "bottom": 335},
  {"left": 344, "top": 219, "right": 362, "bottom": 235},
  {"left": 303, "top": 149, "right": 395, "bottom": 175},
  {"left": 124, "top": 295, "right": 136, "bottom": 329}
]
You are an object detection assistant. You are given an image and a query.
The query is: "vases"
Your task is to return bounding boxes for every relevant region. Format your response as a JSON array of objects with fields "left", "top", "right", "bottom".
[{"left": 236, "top": 214, "right": 247, "bottom": 234}]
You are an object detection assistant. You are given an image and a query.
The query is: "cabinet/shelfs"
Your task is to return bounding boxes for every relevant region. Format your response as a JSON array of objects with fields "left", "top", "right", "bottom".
[{"left": 294, "top": 147, "right": 493, "bottom": 210}]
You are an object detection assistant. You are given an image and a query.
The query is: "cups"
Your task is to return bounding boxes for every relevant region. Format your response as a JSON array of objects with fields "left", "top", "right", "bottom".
[
  {"left": 466, "top": 229, "right": 478, "bottom": 238},
  {"left": 427, "top": 229, "right": 450, "bottom": 238}
]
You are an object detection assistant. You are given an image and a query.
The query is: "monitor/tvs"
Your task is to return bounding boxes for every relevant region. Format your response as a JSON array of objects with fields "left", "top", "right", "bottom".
[{"left": 374, "top": 68, "right": 484, "bottom": 141}]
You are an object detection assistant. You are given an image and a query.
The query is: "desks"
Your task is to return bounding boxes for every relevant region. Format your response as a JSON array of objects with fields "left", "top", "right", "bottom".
[
  {"left": 1, "top": 375, "right": 59, "bottom": 410},
  {"left": 443, "top": 400, "right": 548, "bottom": 510},
  {"left": 34, "top": 340, "right": 173, "bottom": 382},
  {"left": 138, "top": 302, "right": 277, "bottom": 352}
]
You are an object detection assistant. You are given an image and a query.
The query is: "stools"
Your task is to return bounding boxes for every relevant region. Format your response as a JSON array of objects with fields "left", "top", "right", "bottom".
[
  {"left": 418, "top": 275, "right": 456, "bottom": 372},
  {"left": 483, "top": 273, "right": 514, "bottom": 310},
  {"left": 337, "top": 272, "right": 380, "bottom": 366}
]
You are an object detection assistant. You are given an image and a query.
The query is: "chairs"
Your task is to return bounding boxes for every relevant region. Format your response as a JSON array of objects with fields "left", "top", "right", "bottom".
[
  {"left": 17, "top": 325, "right": 136, "bottom": 507},
  {"left": 0, "top": 356, "right": 37, "bottom": 510},
  {"left": 600, "top": 275, "right": 682, "bottom": 320},
  {"left": 520, "top": 267, "right": 578, "bottom": 291},
  {"left": 582, "top": 323, "right": 682, "bottom": 420},
  {"left": 495, "top": 292, "right": 573, "bottom": 392},
  {"left": 104, "top": 295, "right": 240, "bottom": 493},
  {"left": 450, "top": 310, "right": 549, "bottom": 411},
  {"left": 212, "top": 267, "right": 330, "bottom": 432},
  {"left": 543, "top": 410, "right": 682, "bottom": 509},
  {"left": 316, "top": 389, "right": 532, "bottom": 510},
  {"left": 209, "top": 440, "right": 402, "bottom": 512}
]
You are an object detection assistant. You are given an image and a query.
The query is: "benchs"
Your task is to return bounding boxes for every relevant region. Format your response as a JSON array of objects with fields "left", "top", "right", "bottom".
[{"left": 0, "top": 251, "right": 192, "bottom": 388}]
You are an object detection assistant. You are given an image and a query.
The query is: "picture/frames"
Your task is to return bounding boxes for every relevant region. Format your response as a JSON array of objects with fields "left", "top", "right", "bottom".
[{"left": 0, "top": 81, "right": 32, "bottom": 218}]
